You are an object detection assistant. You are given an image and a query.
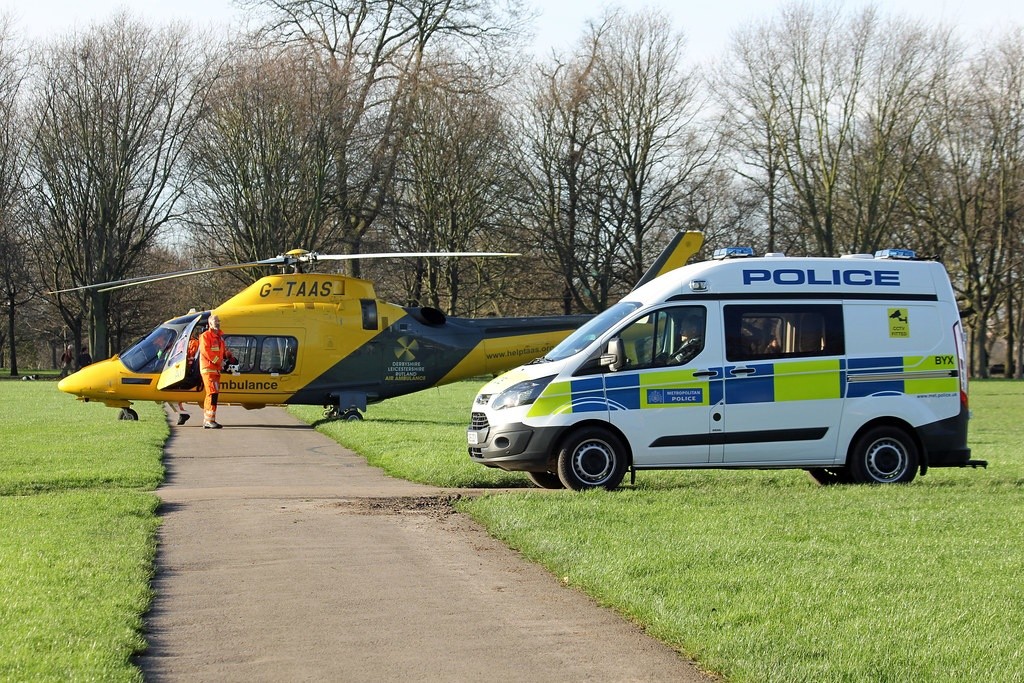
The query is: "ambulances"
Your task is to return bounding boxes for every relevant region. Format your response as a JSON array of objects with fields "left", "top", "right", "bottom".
[{"left": 465, "top": 247, "right": 989, "bottom": 493}]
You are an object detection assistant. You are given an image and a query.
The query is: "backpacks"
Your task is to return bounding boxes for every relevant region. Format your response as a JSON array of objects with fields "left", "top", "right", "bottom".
[{"left": 60, "top": 353, "right": 65, "bottom": 362}]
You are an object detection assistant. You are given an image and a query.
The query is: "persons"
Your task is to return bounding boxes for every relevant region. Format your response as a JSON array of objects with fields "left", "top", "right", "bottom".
[
  {"left": 157, "top": 315, "right": 236, "bottom": 429},
  {"left": 672, "top": 314, "right": 703, "bottom": 362},
  {"left": 764, "top": 339, "right": 781, "bottom": 353},
  {"left": 61, "top": 344, "right": 93, "bottom": 402}
]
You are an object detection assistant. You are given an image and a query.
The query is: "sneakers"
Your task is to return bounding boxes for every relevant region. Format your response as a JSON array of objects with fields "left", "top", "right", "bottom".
[
  {"left": 176, "top": 414, "right": 190, "bottom": 425},
  {"left": 203, "top": 422, "right": 223, "bottom": 429}
]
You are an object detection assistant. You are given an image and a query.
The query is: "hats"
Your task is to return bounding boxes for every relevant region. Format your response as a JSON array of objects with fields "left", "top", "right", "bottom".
[
  {"left": 81, "top": 347, "right": 86, "bottom": 352},
  {"left": 68, "top": 345, "right": 73, "bottom": 350}
]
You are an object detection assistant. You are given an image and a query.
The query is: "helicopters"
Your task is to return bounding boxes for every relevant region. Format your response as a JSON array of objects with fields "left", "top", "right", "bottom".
[{"left": 43, "top": 231, "right": 702, "bottom": 422}]
